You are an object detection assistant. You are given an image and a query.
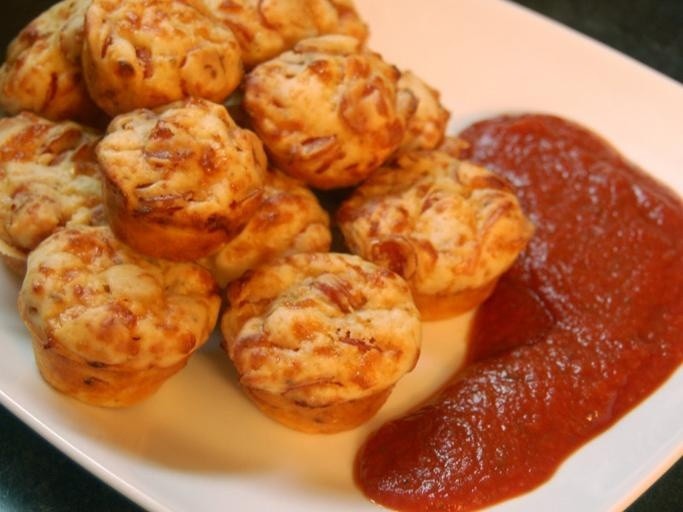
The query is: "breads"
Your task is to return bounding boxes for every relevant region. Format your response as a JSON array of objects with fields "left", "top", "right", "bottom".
[
  {"left": 0, "top": 0, "right": 451, "bottom": 288},
  {"left": 220, "top": 253, "right": 420, "bottom": 434},
  {"left": 337, "top": 148, "right": 535, "bottom": 322},
  {"left": 17, "top": 203, "right": 221, "bottom": 408}
]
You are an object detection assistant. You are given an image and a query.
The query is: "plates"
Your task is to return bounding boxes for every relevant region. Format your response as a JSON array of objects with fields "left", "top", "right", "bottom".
[{"left": 1, "top": 0, "right": 683, "bottom": 512}]
